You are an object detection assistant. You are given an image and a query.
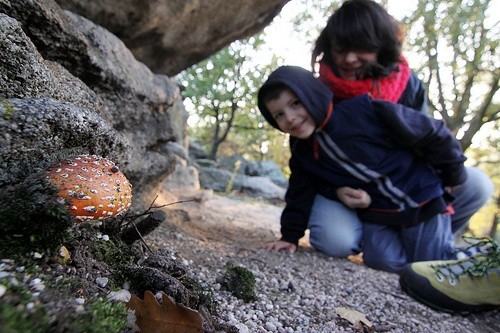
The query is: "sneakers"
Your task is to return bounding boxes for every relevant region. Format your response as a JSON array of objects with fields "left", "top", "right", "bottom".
[{"left": 401, "top": 246, "right": 500, "bottom": 314}]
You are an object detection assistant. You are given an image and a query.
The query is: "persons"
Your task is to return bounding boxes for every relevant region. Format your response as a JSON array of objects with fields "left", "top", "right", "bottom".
[
  {"left": 400, "top": 234, "right": 500, "bottom": 316},
  {"left": 306, "top": 1, "right": 493, "bottom": 258},
  {"left": 257, "top": 64, "right": 467, "bottom": 272}
]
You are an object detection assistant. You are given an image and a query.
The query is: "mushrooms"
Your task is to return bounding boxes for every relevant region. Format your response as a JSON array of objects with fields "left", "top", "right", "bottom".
[{"left": 44, "top": 154, "right": 133, "bottom": 224}]
